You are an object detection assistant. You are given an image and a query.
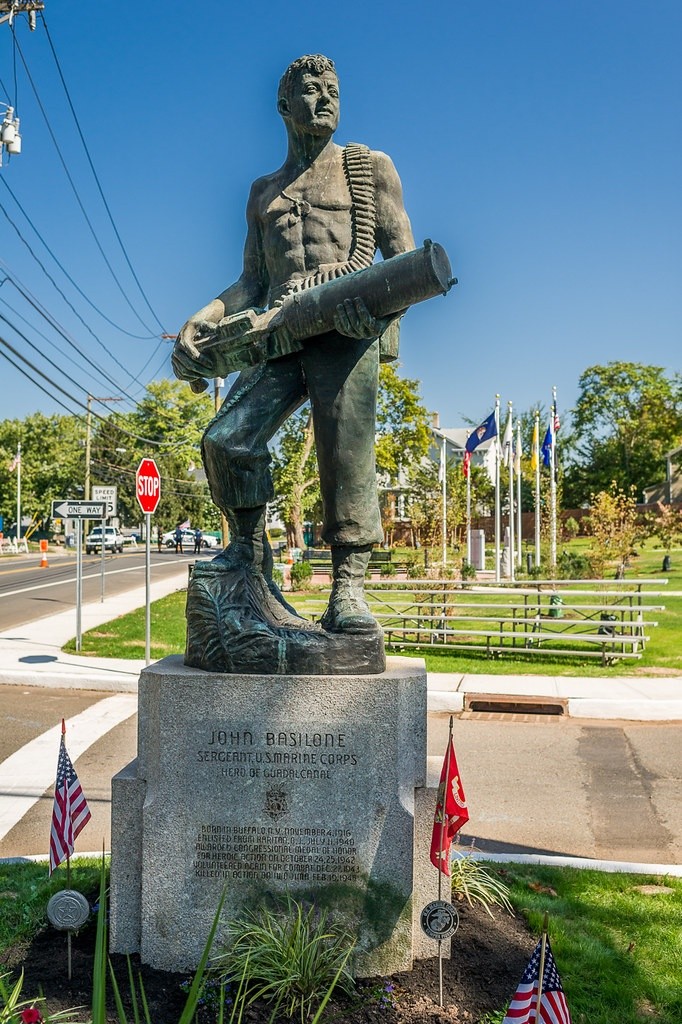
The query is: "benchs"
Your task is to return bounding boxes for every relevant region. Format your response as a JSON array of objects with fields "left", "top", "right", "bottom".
[{"left": 300, "top": 578, "right": 670, "bottom": 666}]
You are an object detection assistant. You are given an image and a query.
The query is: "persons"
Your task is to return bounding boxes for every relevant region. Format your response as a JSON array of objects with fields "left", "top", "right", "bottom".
[
  {"left": 156, "top": 527, "right": 165, "bottom": 552},
  {"left": 192, "top": 528, "right": 203, "bottom": 554},
  {"left": 172, "top": 54, "right": 420, "bottom": 634},
  {"left": 172, "top": 526, "right": 185, "bottom": 554}
]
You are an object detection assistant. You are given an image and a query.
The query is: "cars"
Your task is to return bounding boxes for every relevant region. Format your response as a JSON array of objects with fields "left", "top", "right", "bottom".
[{"left": 158, "top": 528, "right": 221, "bottom": 549}]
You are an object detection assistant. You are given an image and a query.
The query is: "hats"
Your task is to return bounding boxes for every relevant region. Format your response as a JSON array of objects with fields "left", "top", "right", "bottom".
[{"left": 196, "top": 528, "right": 200, "bottom": 530}]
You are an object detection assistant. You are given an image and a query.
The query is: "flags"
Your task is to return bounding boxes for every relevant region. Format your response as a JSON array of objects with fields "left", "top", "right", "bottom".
[
  {"left": 465, "top": 410, "right": 497, "bottom": 452},
  {"left": 482, "top": 440, "right": 497, "bottom": 485},
  {"left": 553, "top": 401, "right": 561, "bottom": 431},
  {"left": 501, "top": 936, "right": 571, "bottom": 1024},
  {"left": 463, "top": 451, "right": 472, "bottom": 477},
  {"left": 530, "top": 424, "right": 538, "bottom": 470},
  {"left": 502, "top": 419, "right": 512, "bottom": 466},
  {"left": 513, "top": 438, "right": 522, "bottom": 474},
  {"left": 430, "top": 734, "right": 469, "bottom": 877},
  {"left": 541, "top": 424, "right": 552, "bottom": 467},
  {"left": 439, "top": 447, "right": 444, "bottom": 481},
  {"left": 48, "top": 736, "right": 91, "bottom": 876}
]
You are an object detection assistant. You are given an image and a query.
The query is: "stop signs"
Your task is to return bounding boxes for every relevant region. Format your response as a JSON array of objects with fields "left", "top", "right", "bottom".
[{"left": 135, "top": 458, "right": 161, "bottom": 515}]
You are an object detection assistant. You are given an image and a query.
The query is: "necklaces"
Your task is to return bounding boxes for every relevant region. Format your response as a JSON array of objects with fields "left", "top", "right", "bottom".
[{"left": 274, "top": 156, "right": 333, "bottom": 218}]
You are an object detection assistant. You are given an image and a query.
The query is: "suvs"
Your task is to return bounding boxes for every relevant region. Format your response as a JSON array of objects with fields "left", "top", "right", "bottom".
[{"left": 85, "top": 526, "right": 125, "bottom": 555}]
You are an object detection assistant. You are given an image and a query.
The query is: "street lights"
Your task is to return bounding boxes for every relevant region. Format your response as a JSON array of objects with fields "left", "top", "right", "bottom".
[{"left": 84, "top": 446, "right": 126, "bottom": 548}]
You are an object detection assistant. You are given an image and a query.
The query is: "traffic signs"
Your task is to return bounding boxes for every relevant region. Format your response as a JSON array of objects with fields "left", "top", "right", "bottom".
[{"left": 52, "top": 501, "right": 108, "bottom": 520}]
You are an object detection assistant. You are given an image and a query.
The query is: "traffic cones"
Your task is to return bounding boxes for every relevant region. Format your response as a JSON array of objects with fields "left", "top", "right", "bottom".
[
  {"left": 38, "top": 552, "right": 49, "bottom": 568},
  {"left": 286, "top": 552, "right": 294, "bottom": 564}
]
